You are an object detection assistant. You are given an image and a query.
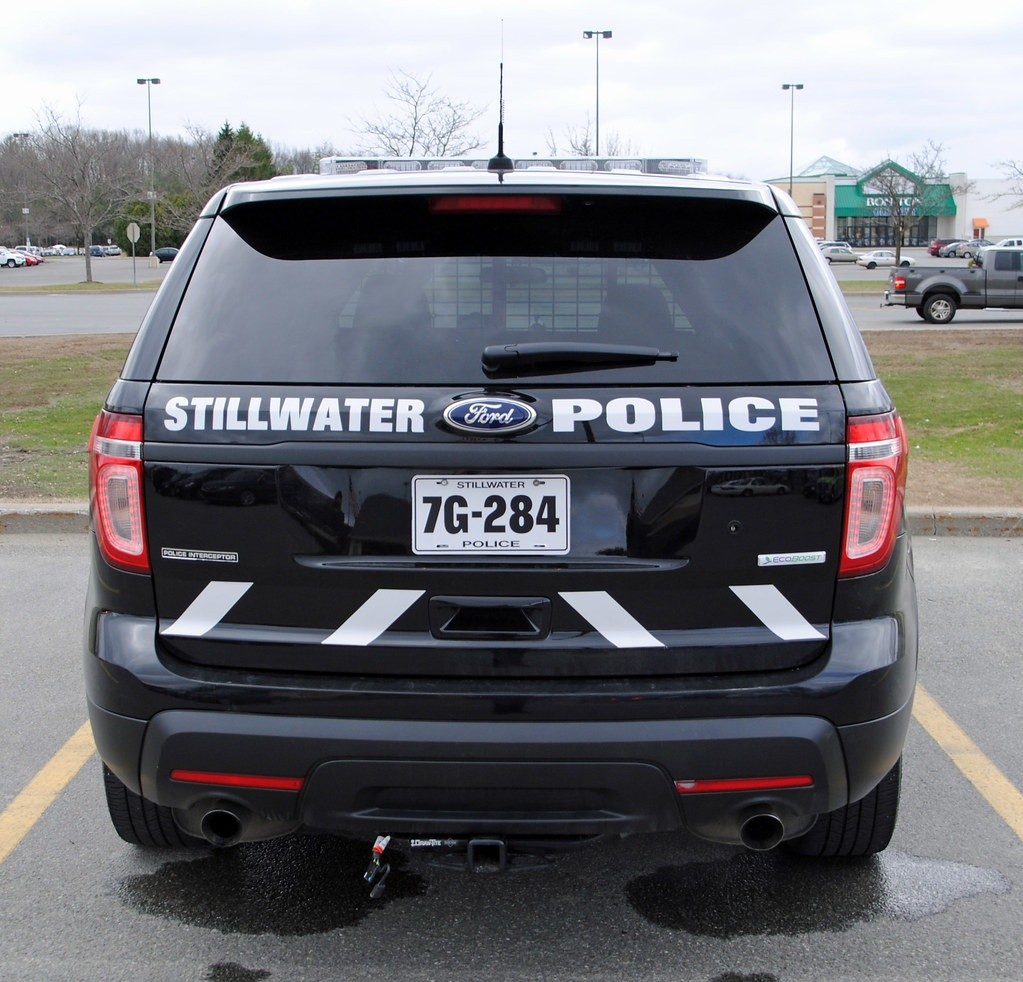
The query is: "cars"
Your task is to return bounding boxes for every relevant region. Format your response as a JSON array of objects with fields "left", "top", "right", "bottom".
[
  {"left": 856, "top": 251, "right": 916, "bottom": 269},
  {"left": 928, "top": 237, "right": 1023, "bottom": 269},
  {"left": 817, "top": 240, "right": 860, "bottom": 264},
  {"left": 0, "top": 244, "right": 122, "bottom": 268},
  {"left": 81, "top": 157, "right": 923, "bottom": 903},
  {"left": 155, "top": 247, "right": 180, "bottom": 263}
]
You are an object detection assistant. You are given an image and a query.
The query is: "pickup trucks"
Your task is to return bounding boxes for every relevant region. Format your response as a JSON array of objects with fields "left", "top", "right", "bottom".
[{"left": 880, "top": 249, "right": 1023, "bottom": 323}]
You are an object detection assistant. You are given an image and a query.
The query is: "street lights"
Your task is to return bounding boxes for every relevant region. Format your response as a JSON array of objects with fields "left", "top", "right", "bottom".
[
  {"left": 136, "top": 78, "right": 160, "bottom": 267},
  {"left": 13, "top": 133, "right": 31, "bottom": 253},
  {"left": 781, "top": 84, "right": 804, "bottom": 196},
  {"left": 582, "top": 30, "right": 612, "bottom": 156}
]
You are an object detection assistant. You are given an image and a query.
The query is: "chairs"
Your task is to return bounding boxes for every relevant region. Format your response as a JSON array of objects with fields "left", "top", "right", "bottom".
[
  {"left": 335, "top": 275, "right": 455, "bottom": 379},
  {"left": 594, "top": 282, "right": 699, "bottom": 374}
]
